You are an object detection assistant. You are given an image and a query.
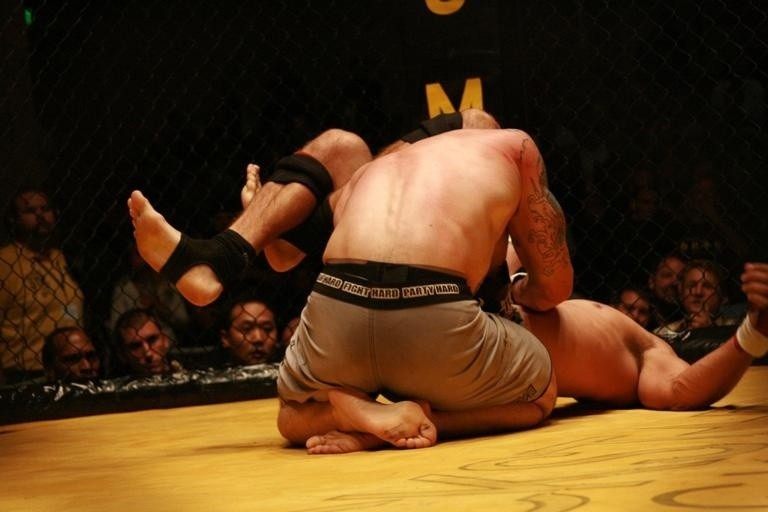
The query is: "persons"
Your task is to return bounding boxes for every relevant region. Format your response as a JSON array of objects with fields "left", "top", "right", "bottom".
[
  {"left": 584, "top": 179, "right": 749, "bottom": 337},
  {"left": 1, "top": 178, "right": 337, "bottom": 403},
  {"left": 276, "top": 126, "right": 577, "bottom": 457},
  {"left": 126, "top": 108, "right": 768, "bottom": 422}
]
